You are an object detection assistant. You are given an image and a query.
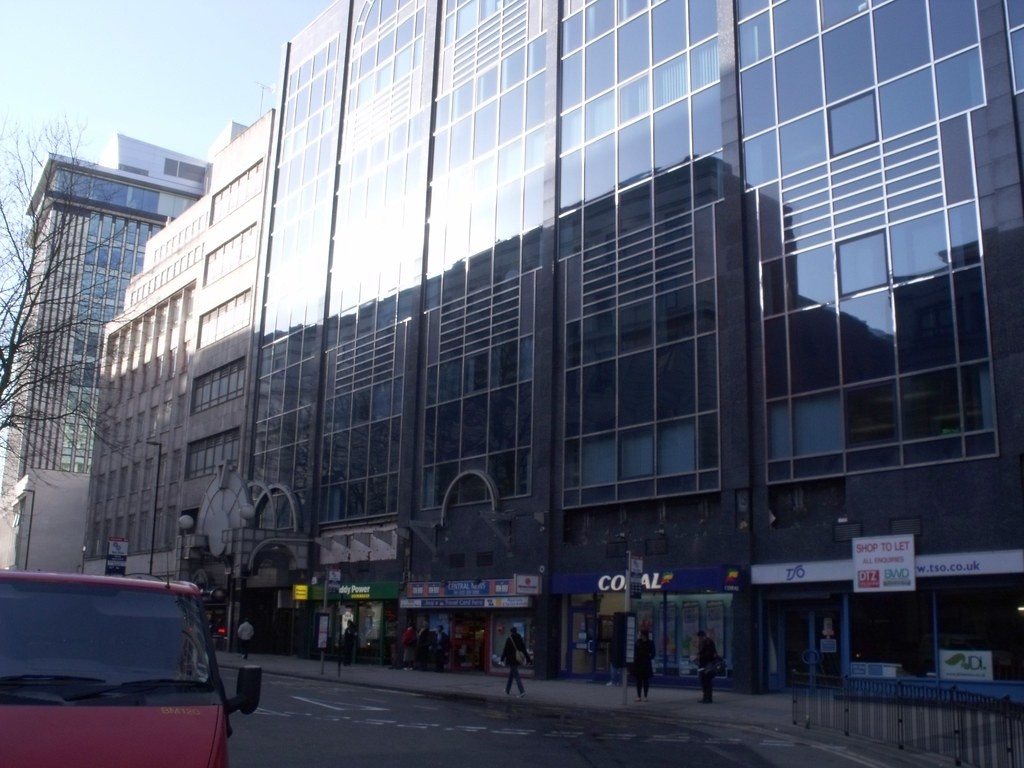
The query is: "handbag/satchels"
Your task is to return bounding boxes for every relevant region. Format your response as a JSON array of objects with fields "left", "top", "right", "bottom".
[
  {"left": 703, "top": 653, "right": 727, "bottom": 679},
  {"left": 515, "top": 650, "right": 527, "bottom": 666}
]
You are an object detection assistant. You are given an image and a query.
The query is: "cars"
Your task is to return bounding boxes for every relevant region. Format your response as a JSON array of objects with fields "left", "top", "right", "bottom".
[{"left": 0, "top": 567, "right": 261, "bottom": 768}]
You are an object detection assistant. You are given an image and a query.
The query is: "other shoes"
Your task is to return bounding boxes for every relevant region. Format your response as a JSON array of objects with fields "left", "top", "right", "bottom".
[
  {"left": 636, "top": 697, "right": 641, "bottom": 702},
  {"left": 517, "top": 692, "right": 528, "bottom": 699},
  {"left": 504, "top": 689, "right": 511, "bottom": 697},
  {"left": 644, "top": 697, "right": 648, "bottom": 702},
  {"left": 698, "top": 700, "right": 710, "bottom": 704}
]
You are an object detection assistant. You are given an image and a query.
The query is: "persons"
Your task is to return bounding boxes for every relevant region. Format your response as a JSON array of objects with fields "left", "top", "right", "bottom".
[
  {"left": 342, "top": 619, "right": 359, "bottom": 666},
  {"left": 634, "top": 630, "right": 656, "bottom": 701},
  {"left": 605, "top": 663, "right": 620, "bottom": 687},
  {"left": 237, "top": 617, "right": 254, "bottom": 660},
  {"left": 498, "top": 627, "right": 532, "bottom": 697},
  {"left": 697, "top": 630, "right": 725, "bottom": 703},
  {"left": 401, "top": 621, "right": 450, "bottom": 673}
]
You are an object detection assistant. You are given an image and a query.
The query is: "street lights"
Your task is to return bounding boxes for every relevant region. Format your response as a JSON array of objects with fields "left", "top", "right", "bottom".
[
  {"left": 146, "top": 440, "right": 163, "bottom": 574},
  {"left": 23, "top": 488, "right": 36, "bottom": 569}
]
